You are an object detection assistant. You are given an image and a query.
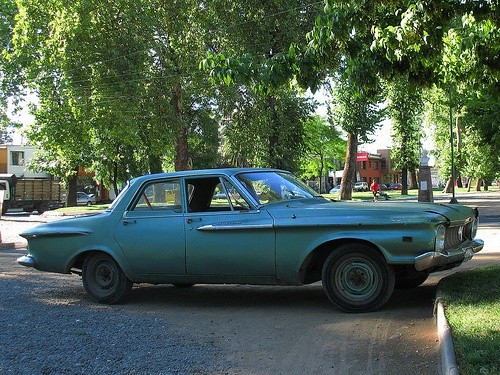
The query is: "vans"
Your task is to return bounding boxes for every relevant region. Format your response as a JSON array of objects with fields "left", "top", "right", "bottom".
[{"left": 353, "top": 181, "right": 369, "bottom": 191}]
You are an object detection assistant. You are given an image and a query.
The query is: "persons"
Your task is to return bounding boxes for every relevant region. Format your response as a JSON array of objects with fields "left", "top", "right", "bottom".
[
  {"left": 421, "top": 150, "right": 430, "bottom": 166},
  {"left": 377, "top": 190, "right": 391, "bottom": 200},
  {"left": 370, "top": 179, "right": 378, "bottom": 202},
  {"left": 283, "top": 185, "right": 290, "bottom": 199},
  {"left": 437, "top": 181, "right": 441, "bottom": 187}
]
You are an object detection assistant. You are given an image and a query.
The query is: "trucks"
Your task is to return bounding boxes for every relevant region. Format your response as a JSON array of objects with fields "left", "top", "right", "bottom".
[{"left": 0, "top": 174, "right": 63, "bottom": 215}]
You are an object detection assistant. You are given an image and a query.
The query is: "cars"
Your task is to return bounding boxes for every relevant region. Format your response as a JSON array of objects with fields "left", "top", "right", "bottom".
[
  {"left": 60, "top": 192, "right": 97, "bottom": 207},
  {"left": 389, "top": 183, "right": 402, "bottom": 190},
  {"left": 379, "top": 184, "right": 387, "bottom": 190},
  {"left": 16, "top": 169, "right": 485, "bottom": 315},
  {"left": 330, "top": 185, "right": 341, "bottom": 193},
  {"left": 432, "top": 184, "right": 439, "bottom": 189}
]
